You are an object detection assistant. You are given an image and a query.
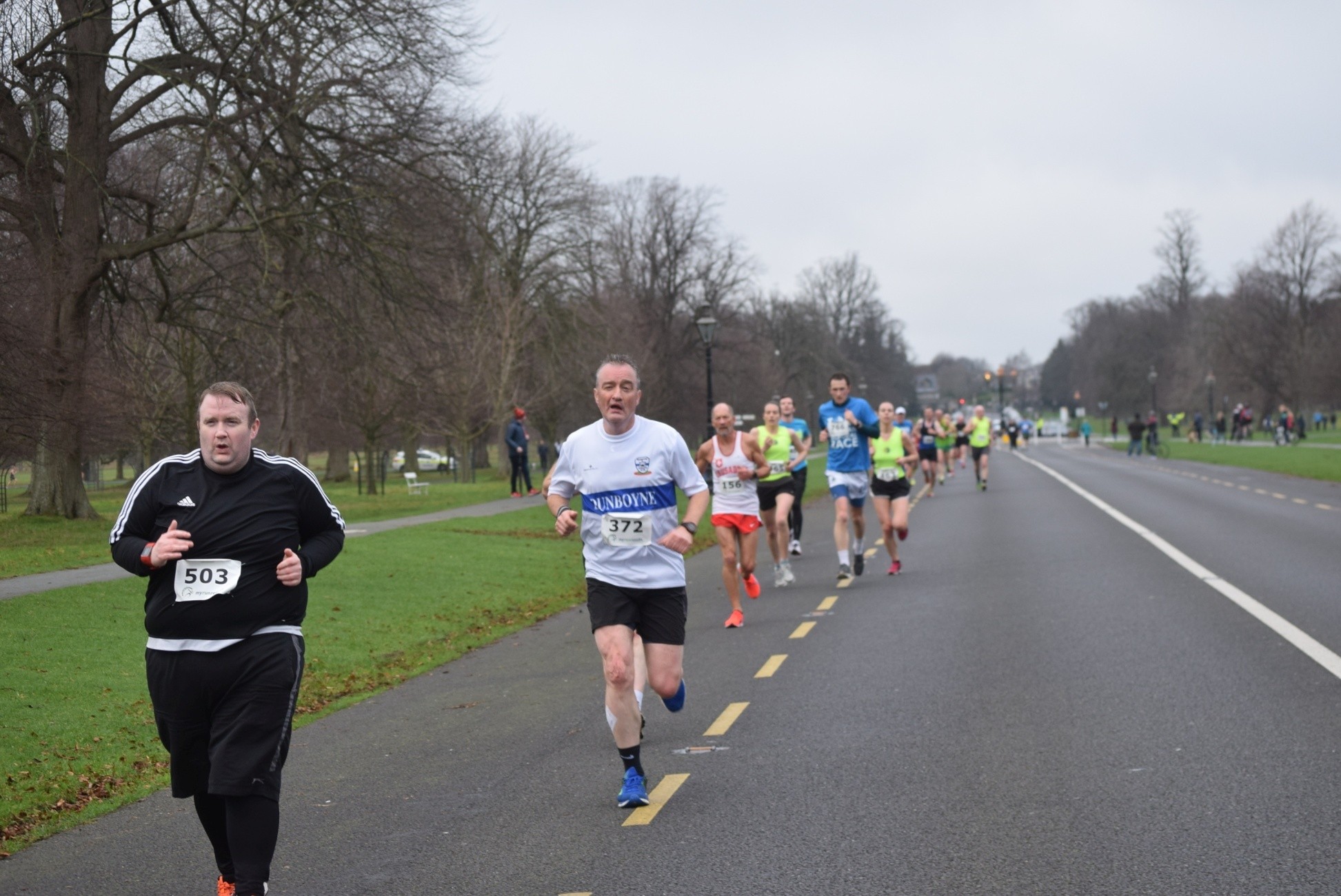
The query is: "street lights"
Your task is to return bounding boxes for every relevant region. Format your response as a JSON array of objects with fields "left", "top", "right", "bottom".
[
  {"left": 1205, "top": 371, "right": 1215, "bottom": 435},
  {"left": 1148, "top": 365, "right": 1157, "bottom": 416},
  {"left": 984, "top": 367, "right": 1016, "bottom": 433},
  {"left": 694, "top": 305, "right": 717, "bottom": 496}
]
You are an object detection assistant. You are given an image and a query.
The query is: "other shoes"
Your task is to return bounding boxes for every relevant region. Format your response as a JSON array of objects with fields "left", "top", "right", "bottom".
[{"left": 640, "top": 715, "right": 647, "bottom": 741}]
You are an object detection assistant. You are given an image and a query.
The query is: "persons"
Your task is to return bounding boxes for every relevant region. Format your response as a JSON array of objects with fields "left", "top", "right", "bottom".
[
  {"left": 817, "top": 374, "right": 881, "bottom": 579},
  {"left": 893, "top": 401, "right": 1341, "bottom": 497},
  {"left": 107, "top": 380, "right": 346, "bottom": 896},
  {"left": 547, "top": 352, "right": 711, "bottom": 807},
  {"left": 504, "top": 406, "right": 541, "bottom": 498},
  {"left": 537, "top": 439, "right": 549, "bottom": 470},
  {"left": 554, "top": 437, "right": 563, "bottom": 458},
  {"left": 749, "top": 401, "right": 809, "bottom": 588},
  {"left": 541, "top": 460, "right": 648, "bottom": 740},
  {"left": 866, "top": 401, "right": 920, "bottom": 576},
  {"left": 695, "top": 403, "right": 774, "bottom": 628},
  {"left": 778, "top": 396, "right": 814, "bottom": 555}
]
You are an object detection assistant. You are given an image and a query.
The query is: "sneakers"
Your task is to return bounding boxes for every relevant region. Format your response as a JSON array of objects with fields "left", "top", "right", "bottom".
[
  {"left": 736, "top": 563, "right": 760, "bottom": 598},
  {"left": 510, "top": 492, "right": 521, "bottom": 498},
  {"left": 660, "top": 678, "right": 686, "bottom": 712},
  {"left": 216, "top": 874, "right": 271, "bottom": 896},
  {"left": 528, "top": 489, "right": 541, "bottom": 496},
  {"left": 836, "top": 564, "right": 851, "bottom": 579},
  {"left": 899, "top": 529, "right": 908, "bottom": 541},
  {"left": 725, "top": 610, "right": 745, "bottom": 629},
  {"left": 788, "top": 528, "right": 801, "bottom": 555},
  {"left": 888, "top": 559, "right": 903, "bottom": 576},
  {"left": 853, "top": 553, "right": 865, "bottom": 576},
  {"left": 617, "top": 767, "right": 649, "bottom": 808},
  {"left": 775, "top": 562, "right": 795, "bottom": 589},
  {"left": 906, "top": 438, "right": 999, "bottom": 498}
]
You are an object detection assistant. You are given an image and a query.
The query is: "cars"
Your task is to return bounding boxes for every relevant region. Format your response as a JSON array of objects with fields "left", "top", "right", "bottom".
[
  {"left": 392, "top": 449, "right": 454, "bottom": 472},
  {"left": 1034, "top": 423, "right": 1072, "bottom": 436}
]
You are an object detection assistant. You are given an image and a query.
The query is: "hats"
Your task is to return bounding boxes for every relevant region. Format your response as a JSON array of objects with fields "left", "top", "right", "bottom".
[
  {"left": 514, "top": 408, "right": 525, "bottom": 419},
  {"left": 895, "top": 406, "right": 906, "bottom": 415}
]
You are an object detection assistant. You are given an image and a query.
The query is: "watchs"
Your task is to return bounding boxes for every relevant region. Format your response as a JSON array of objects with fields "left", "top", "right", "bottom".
[
  {"left": 680, "top": 522, "right": 697, "bottom": 535},
  {"left": 140, "top": 542, "right": 161, "bottom": 569},
  {"left": 854, "top": 419, "right": 861, "bottom": 428},
  {"left": 751, "top": 469, "right": 757, "bottom": 479}
]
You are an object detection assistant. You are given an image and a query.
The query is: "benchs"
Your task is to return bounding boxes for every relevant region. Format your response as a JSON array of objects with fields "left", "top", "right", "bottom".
[{"left": 403, "top": 471, "right": 429, "bottom": 495}]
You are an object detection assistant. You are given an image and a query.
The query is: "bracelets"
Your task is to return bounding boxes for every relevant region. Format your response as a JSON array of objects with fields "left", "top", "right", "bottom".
[{"left": 556, "top": 505, "right": 571, "bottom": 519}]
